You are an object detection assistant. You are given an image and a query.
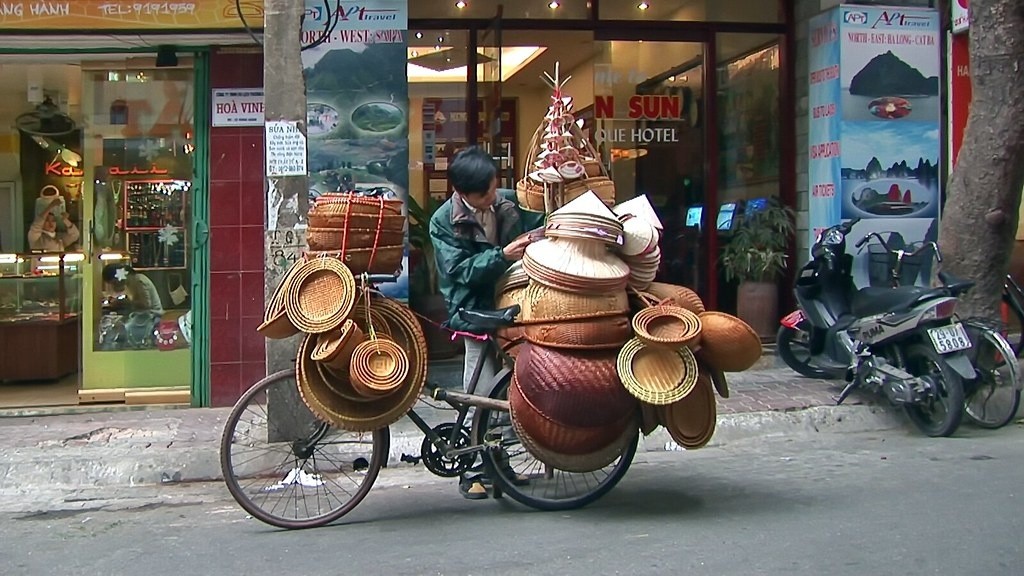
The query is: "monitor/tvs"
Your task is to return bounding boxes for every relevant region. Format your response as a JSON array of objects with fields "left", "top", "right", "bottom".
[
  {"left": 684, "top": 203, "right": 704, "bottom": 233},
  {"left": 716, "top": 200, "right": 743, "bottom": 237},
  {"left": 744, "top": 196, "right": 769, "bottom": 221}
]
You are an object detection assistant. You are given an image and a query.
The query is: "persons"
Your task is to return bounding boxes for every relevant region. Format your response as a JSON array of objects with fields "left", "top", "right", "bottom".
[
  {"left": 27, "top": 199, "right": 80, "bottom": 254},
  {"left": 102, "top": 263, "right": 162, "bottom": 315},
  {"left": 429, "top": 146, "right": 545, "bottom": 500}
]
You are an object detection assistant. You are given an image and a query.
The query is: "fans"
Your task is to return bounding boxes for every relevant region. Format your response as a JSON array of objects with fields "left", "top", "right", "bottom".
[{"left": 15, "top": 95, "right": 76, "bottom": 136}]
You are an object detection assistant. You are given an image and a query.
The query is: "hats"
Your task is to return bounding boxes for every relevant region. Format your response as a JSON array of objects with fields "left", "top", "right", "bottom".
[{"left": 521, "top": 190, "right": 662, "bottom": 296}]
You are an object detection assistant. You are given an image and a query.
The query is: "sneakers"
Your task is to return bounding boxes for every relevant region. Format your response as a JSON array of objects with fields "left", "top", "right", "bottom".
[
  {"left": 512, "top": 472, "right": 529, "bottom": 485},
  {"left": 459, "top": 481, "right": 488, "bottom": 499}
]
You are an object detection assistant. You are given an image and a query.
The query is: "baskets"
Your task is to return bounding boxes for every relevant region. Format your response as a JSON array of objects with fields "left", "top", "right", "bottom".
[
  {"left": 493, "top": 283, "right": 762, "bottom": 473},
  {"left": 868, "top": 242, "right": 925, "bottom": 289},
  {"left": 256, "top": 191, "right": 429, "bottom": 434},
  {"left": 508, "top": 152, "right": 618, "bottom": 213}
]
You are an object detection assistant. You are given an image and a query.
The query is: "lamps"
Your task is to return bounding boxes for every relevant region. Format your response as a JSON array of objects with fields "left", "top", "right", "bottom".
[{"left": 154, "top": 43, "right": 180, "bottom": 67}]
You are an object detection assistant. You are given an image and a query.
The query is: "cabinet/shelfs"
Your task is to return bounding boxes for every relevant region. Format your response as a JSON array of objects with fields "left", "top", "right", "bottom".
[{"left": 0, "top": 251, "right": 83, "bottom": 383}]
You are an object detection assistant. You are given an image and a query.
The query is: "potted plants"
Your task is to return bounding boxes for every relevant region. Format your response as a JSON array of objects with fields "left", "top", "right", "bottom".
[
  {"left": 407, "top": 194, "right": 461, "bottom": 361},
  {"left": 722, "top": 213, "right": 792, "bottom": 342}
]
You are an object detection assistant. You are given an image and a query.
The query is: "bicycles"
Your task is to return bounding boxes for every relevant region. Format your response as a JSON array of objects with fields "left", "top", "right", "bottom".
[
  {"left": 993, "top": 275, "right": 1024, "bottom": 369},
  {"left": 218, "top": 270, "right": 641, "bottom": 530},
  {"left": 853, "top": 229, "right": 1024, "bottom": 429}
]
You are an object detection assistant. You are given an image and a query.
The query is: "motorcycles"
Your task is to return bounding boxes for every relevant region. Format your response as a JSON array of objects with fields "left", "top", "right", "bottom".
[{"left": 775, "top": 219, "right": 978, "bottom": 437}]
